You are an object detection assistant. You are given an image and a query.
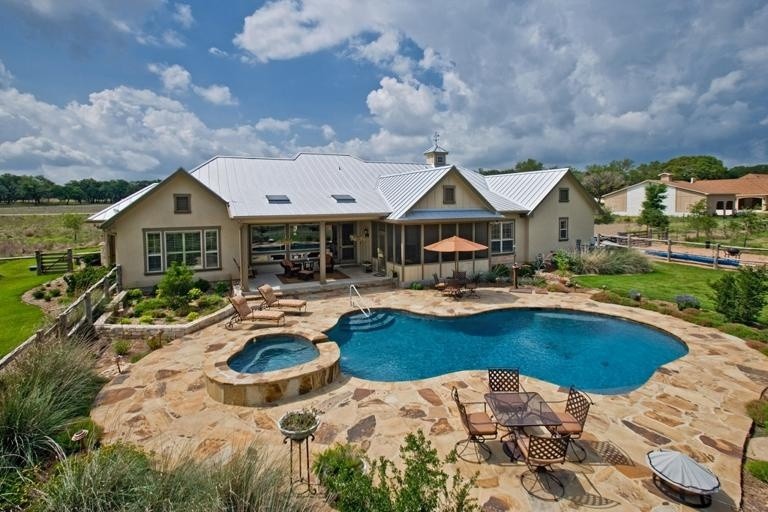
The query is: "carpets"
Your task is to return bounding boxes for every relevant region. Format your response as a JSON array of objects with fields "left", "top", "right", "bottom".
[{"left": 276, "top": 269, "right": 351, "bottom": 284}]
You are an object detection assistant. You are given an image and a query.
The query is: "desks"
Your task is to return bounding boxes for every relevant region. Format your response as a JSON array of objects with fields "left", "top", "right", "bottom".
[{"left": 483, "top": 392, "right": 561, "bottom": 461}]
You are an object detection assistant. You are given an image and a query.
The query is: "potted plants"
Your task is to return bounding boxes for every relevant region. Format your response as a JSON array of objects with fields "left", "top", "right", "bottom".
[{"left": 279, "top": 409, "right": 321, "bottom": 440}]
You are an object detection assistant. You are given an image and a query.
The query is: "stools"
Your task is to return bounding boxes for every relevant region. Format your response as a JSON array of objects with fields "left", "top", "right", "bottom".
[{"left": 297, "top": 270, "right": 316, "bottom": 280}]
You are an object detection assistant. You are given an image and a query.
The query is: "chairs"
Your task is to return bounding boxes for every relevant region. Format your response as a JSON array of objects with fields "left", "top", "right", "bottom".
[
  {"left": 450, "top": 386, "right": 498, "bottom": 464},
  {"left": 538, "top": 384, "right": 594, "bottom": 463},
  {"left": 225, "top": 295, "right": 285, "bottom": 331},
  {"left": 317, "top": 254, "right": 335, "bottom": 273},
  {"left": 516, "top": 433, "right": 572, "bottom": 502},
  {"left": 487, "top": 367, "right": 529, "bottom": 431},
  {"left": 280, "top": 259, "right": 302, "bottom": 278},
  {"left": 433, "top": 271, "right": 479, "bottom": 302},
  {"left": 251, "top": 284, "right": 307, "bottom": 316}
]
